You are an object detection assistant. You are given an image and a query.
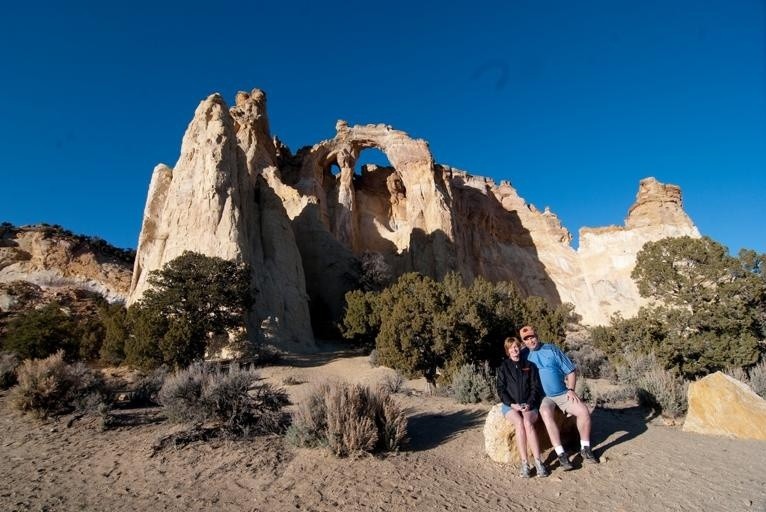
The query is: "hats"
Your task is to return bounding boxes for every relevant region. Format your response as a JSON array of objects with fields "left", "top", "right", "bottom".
[{"left": 519, "top": 326, "right": 534, "bottom": 340}]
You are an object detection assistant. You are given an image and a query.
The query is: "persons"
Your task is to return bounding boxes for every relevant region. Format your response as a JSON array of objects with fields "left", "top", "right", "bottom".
[{"left": 497, "top": 326, "right": 600, "bottom": 477}]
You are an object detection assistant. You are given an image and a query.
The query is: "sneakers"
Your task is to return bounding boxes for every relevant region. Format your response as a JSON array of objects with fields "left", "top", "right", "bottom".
[
  {"left": 558, "top": 452, "right": 575, "bottom": 471},
  {"left": 580, "top": 446, "right": 600, "bottom": 464},
  {"left": 520, "top": 459, "right": 531, "bottom": 478},
  {"left": 534, "top": 461, "right": 548, "bottom": 477}
]
